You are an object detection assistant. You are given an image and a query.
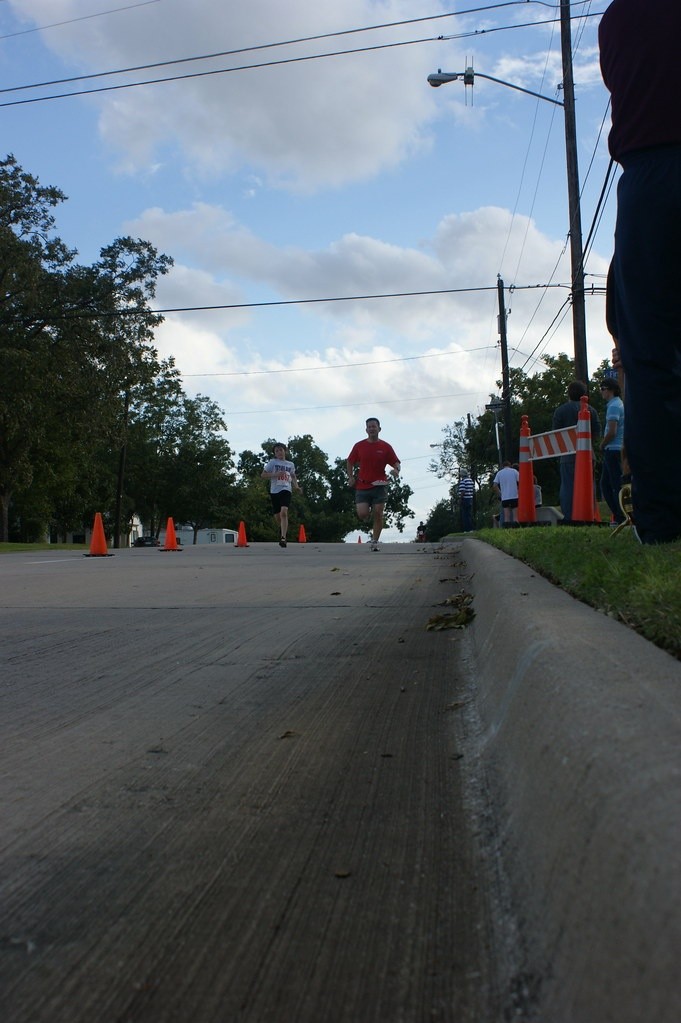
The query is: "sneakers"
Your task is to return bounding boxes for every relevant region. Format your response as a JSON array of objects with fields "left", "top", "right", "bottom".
[
  {"left": 369, "top": 541, "right": 380, "bottom": 551},
  {"left": 364, "top": 507, "right": 372, "bottom": 523}
]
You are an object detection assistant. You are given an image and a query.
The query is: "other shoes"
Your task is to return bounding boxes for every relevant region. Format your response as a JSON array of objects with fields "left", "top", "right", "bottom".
[{"left": 279, "top": 541, "right": 287, "bottom": 548}]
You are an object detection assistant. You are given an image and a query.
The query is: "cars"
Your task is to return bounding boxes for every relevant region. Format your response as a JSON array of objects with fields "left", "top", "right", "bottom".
[{"left": 130, "top": 536, "right": 161, "bottom": 547}]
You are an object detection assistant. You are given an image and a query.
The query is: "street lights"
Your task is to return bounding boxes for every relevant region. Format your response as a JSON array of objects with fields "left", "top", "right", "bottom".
[
  {"left": 429, "top": 443, "right": 477, "bottom": 519},
  {"left": 424, "top": 67, "right": 600, "bottom": 499}
]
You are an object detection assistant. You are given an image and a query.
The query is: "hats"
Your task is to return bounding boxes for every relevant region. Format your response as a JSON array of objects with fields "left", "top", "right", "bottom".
[{"left": 459, "top": 469, "right": 469, "bottom": 476}]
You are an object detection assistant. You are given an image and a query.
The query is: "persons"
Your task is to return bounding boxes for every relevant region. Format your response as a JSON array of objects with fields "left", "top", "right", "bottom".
[
  {"left": 600, "top": 379, "right": 625, "bottom": 525},
  {"left": 492, "top": 460, "right": 542, "bottom": 528},
  {"left": 458, "top": 470, "right": 475, "bottom": 533},
  {"left": 552, "top": 381, "right": 603, "bottom": 522},
  {"left": 347, "top": 418, "right": 401, "bottom": 551},
  {"left": 417, "top": 521, "right": 426, "bottom": 533},
  {"left": 598, "top": 0, "right": 681, "bottom": 546},
  {"left": 261, "top": 443, "right": 303, "bottom": 547}
]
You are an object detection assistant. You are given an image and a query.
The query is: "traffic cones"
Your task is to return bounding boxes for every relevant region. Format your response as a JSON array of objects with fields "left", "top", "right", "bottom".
[
  {"left": 82, "top": 512, "right": 116, "bottom": 558},
  {"left": 297, "top": 524, "right": 308, "bottom": 544},
  {"left": 594, "top": 503, "right": 602, "bottom": 522},
  {"left": 510, "top": 414, "right": 552, "bottom": 529},
  {"left": 232, "top": 521, "right": 251, "bottom": 548},
  {"left": 158, "top": 517, "right": 184, "bottom": 552},
  {"left": 556, "top": 396, "right": 613, "bottom": 528},
  {"left": 357, "top": 536, "right": 362, "bottom": 544}
]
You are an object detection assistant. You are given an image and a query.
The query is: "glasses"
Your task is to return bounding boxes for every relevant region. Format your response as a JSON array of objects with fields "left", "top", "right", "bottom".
[{"left": 601, "top": 387, "right": 608, "bottom": 390}]
